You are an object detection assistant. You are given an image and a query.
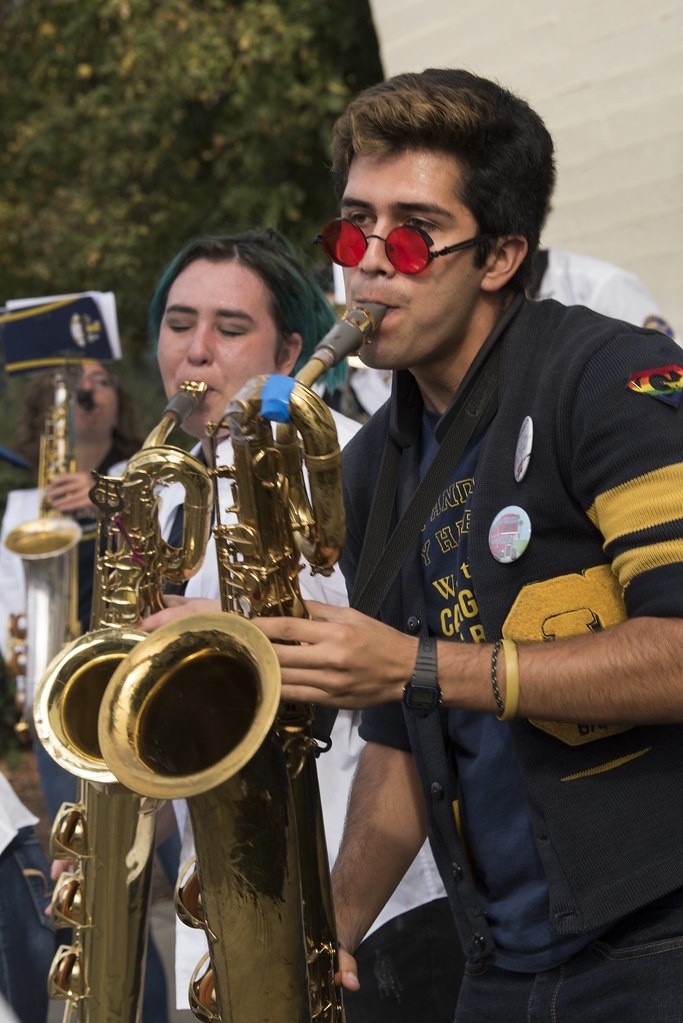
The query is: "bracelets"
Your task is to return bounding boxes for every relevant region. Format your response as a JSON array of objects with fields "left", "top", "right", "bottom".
[{"left": 491, "top": 639, "right": 520, "bottom": 721}]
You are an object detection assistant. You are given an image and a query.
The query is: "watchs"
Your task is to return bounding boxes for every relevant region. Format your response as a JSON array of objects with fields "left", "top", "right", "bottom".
[{"left": 402, "top": 635, "right": 443, "bottom": 711}]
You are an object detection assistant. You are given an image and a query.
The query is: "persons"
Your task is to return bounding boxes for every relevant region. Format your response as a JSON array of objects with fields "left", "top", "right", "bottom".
[
  {"left": 0, "top": 775, "right": 169, "bottom": 1023},
  {"left": 253, "top": 68, "right": 683, "bottom": 1023},
  {"left": 536, "top": 254, "right": 674, "bottom": 344},
  {"left": 0, "top": 363, "right": 144, "bottom": 816},
  {"left": 45, "top": 229, "right": 467, "bottom": 1023}
]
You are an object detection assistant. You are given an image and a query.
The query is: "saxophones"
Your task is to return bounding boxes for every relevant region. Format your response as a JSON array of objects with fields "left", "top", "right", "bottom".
[
  {"left": 98, "top": 298, "right": 385, "bottom": 1022},
  {"left": 30, "top": 378, "right": 207, "bottom": 1022},
  {"left": 0, "top": 373, "right": 80, "bottom": 755}
]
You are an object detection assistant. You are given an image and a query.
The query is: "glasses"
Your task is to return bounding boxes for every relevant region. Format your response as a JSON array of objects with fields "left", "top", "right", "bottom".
[{"left": 319, "top": 218, "right": 477, "bottom": 278}]
